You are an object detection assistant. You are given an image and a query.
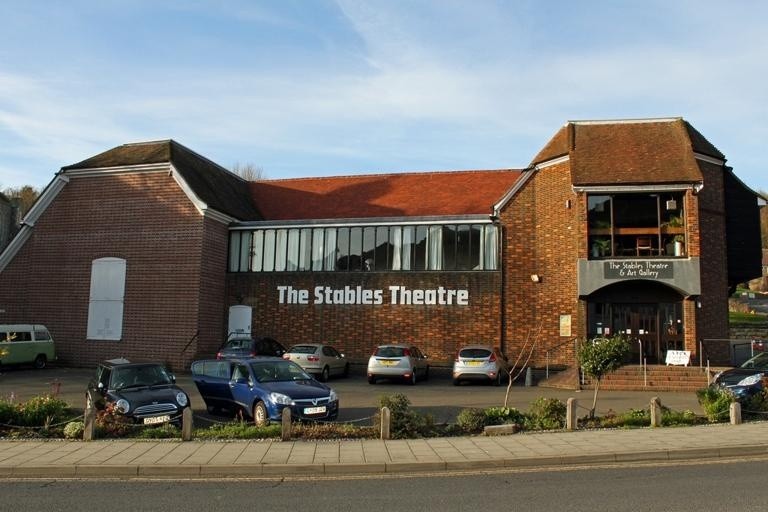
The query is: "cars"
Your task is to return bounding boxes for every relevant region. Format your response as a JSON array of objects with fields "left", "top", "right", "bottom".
[
  {"left": 452, "top": 345, "right": 509, "bottom": 384},
  {"left": 711, "top": 351, "right": 768, "bottom": 416},
  {"left": 190, "top": 337, "right": 350, "bottom": 427},
  {"left": 366, "top": 344, "right": 430, "bottom": 384}
]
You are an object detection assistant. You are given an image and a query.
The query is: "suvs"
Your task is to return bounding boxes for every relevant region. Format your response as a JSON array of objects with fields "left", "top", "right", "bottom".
[{"left": 84, "top": 358, "right": 191, "bottom": 432}]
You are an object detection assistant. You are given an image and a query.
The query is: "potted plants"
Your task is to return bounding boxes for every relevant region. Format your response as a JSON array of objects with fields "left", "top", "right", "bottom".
[
  {"left": 591, "top": 238, "right": 611, "bottom": 258},
  {"left": 664, "top": 216, "right": 684, "bottom": 257}
]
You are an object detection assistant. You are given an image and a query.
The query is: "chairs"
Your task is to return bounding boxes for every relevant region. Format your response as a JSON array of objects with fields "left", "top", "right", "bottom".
[{"left": 632, "top": 236, "right": 652, "bottom": 256}]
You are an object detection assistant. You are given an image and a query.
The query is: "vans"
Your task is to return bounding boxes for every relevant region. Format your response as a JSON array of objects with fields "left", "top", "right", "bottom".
[{"left": 0, "top": 325, "right": 57, "bottom": 371}]
